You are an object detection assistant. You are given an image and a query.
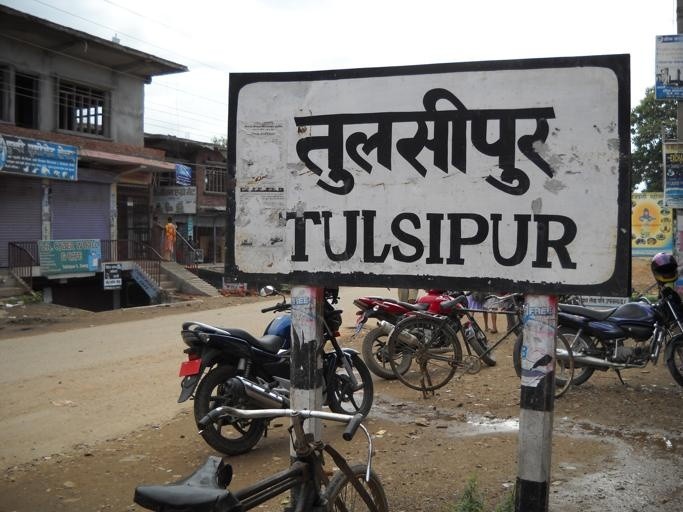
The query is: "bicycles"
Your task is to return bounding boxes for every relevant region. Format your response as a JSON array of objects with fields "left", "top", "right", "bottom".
[
  {"left": 133, "top": 404, "right": 391, "bottom": 512},
  {"left": 385, "top": 291, "right": 576, "bottom": 400}
]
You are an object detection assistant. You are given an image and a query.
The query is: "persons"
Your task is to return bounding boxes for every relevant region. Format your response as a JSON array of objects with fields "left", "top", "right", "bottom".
[
  {"left": 639, "top": 208, "right": 657, "bottom": 238},
  {"left": 194, "top": 240, "right": 201, "bottom": 249},
  {"left": 184, "top": 235, "right": 195, "bottom": 267},
  {"left": 481, "top": 312, "right": 498, "bottom": 333},
  {"left": 150, "top": 216, "right": 165, "bottom": 261},
  {"left": 162, "top": 216, "right": 178, "bottom": 262}
]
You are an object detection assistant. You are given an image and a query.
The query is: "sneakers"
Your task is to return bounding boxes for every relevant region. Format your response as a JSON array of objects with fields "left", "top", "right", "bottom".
[{"left": 491, "top": 329, "right": 497, "bottom": 333}]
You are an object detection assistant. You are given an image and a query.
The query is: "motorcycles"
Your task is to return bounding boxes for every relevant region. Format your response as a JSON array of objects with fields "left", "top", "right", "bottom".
[
  {"left": 176, "top": 284, "right": 375, "bottom": 457},
  {"left": 555, "top": 282, "right": 683, "bottom": 389},
  {"left": 353, "top": 287, "right": 497, "bottom": 381}
]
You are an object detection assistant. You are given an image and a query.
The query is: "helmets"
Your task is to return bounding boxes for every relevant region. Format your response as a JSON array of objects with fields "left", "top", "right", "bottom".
[{"left": 651, "top": 252, "right": 678, "bottom": 281}]
